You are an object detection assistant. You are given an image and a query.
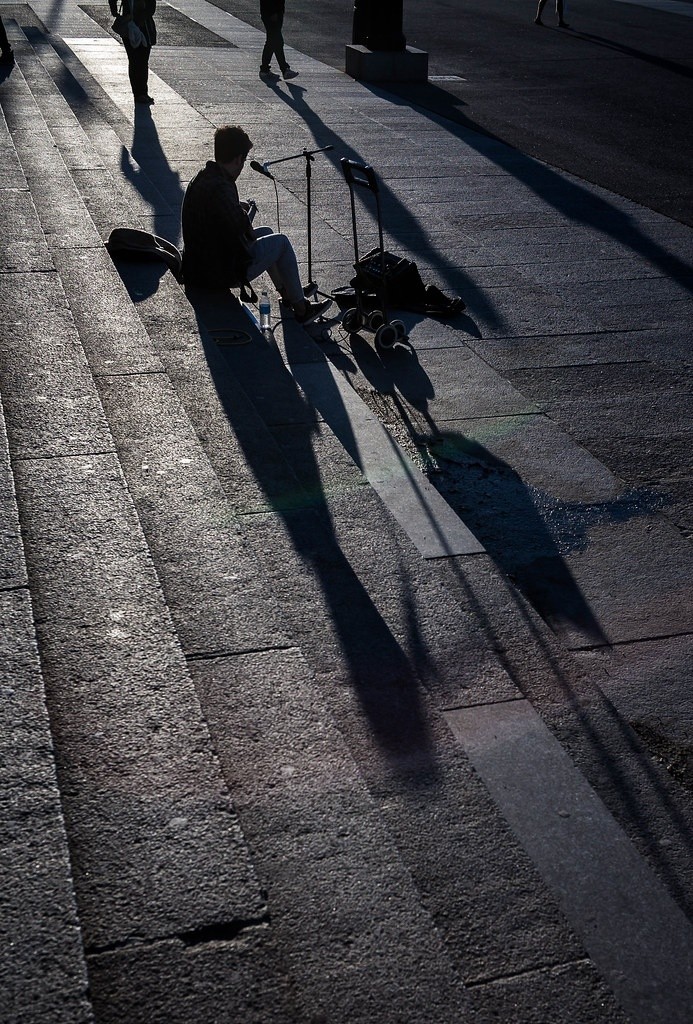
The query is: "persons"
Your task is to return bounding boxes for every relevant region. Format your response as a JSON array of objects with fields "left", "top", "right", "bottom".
[
  {"left": 533, "top": 0, "right": 570, "bottom": 27},
  {"left": 182, "top": 127, "right": 332, "bottom": 326},
  {"left": 0, "top": 16, "right": 15, "bottom": 64},
  {"left": 108, "top": 0, "right": 157, "bottom": 104},
  {"left": 259, "top": 0, "right": 299, "bottom": 80}
]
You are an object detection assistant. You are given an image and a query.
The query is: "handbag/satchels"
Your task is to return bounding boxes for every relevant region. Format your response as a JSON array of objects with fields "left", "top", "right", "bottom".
[
  {"left": 112, "top": 15, "right": 133, "bottom": 38},
  {"left": 106, "top": 226, "right": 183, "bottom": 272}
]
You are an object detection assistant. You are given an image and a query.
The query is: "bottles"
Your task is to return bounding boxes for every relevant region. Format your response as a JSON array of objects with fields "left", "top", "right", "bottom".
[{"left": 260, "top": 292, "right": 271, "bottom": 328}]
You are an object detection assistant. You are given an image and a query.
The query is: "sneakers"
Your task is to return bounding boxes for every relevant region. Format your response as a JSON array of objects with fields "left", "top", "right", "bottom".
[
  {"left": 282, "top": 282, "right": 318, "bottom": 309},
  {"left": 295, "top": 297, "right": 333, "bottom": 326}
]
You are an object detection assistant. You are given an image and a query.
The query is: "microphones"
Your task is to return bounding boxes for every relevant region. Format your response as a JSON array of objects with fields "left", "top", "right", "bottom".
[{"left": 250, "top": 161, "right": 274, "bottom": 181}]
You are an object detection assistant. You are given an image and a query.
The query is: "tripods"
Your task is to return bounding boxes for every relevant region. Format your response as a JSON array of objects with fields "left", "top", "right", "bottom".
[{"left": 263, "top": 143, "right": 336, "bottom": 322}]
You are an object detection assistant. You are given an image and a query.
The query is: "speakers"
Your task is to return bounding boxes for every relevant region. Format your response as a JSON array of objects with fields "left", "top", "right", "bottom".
[{"left": 358, "top": 248, "right": 428, "bottom": 308}]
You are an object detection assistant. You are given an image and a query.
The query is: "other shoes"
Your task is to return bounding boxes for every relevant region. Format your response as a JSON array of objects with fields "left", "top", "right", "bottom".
[
  {"left": 559, "top": 22, "right": 569, "bottom": 27},
  {"left": 134, "top": 93, "right": 154, "bottom": 104},
  {"left": 259, "top": 71, "right": 278, "bottom": 80},
  {"left": 283, "top": 69, "right": 299, "bottom": 79},
  {"left": 534, "top": 19, "right": 543, "bottom": 25}
]
